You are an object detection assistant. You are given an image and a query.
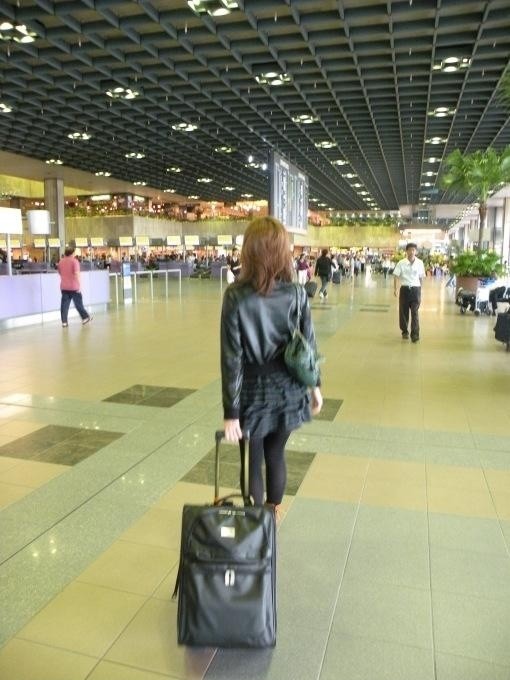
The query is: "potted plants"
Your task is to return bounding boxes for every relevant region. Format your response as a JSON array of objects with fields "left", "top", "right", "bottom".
[{"left": 438, "top": 144, "right": 510, "bottom": 302}]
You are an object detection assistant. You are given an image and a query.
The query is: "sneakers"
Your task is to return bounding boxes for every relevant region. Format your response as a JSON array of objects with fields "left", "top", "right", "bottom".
[
  {"left": 402, "top": 332, "right": 420, "bottom": 343},
  {"left": 82, "top": 317, "right": 90, "bottom": 324},
  {"left": 318, "top": 291, "right": 328, "bottom": 298},
  {"left": 62, "top": 322, "right": 68, "bottom": 327}
]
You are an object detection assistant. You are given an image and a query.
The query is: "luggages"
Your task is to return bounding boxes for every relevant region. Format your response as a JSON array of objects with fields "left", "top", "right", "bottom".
[
  {"left": 333, "top": 272, "right": 341, "bottom": 284},
  {"left": 171, "top": 429, "right": 278, "bottom": 650},
  {"left": 305, "top": 281, "right": 318, "bottom": 297}
]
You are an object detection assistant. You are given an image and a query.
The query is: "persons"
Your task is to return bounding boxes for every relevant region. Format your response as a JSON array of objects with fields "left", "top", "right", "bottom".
[
  {"left": 53, "top": 246, "right": 95, "bottom": 330},
  {"left": 389, "top": 242, "right": 427, "bottom": 346},
  {"left": 219, "top": 214, "right": 324, "bottom": 532}
]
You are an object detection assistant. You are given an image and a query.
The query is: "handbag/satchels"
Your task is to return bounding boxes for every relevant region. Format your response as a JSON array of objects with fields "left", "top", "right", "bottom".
[
  {"left": 456, "top": 291, "right": 476, "bottom": 311},
  {"left": 284, "top": 284, "right": 320, "bottom": 387},
  {"left": 489, "top": 286, "right": 510, "bottom": 301},
  {"left": 495, "top": 312, "right": 510, "bottom": 343}
]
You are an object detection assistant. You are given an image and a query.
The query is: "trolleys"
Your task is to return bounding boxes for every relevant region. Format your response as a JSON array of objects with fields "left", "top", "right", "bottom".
[{"left": 456, "top": 287, "right": 492, "bottom": 316}]
